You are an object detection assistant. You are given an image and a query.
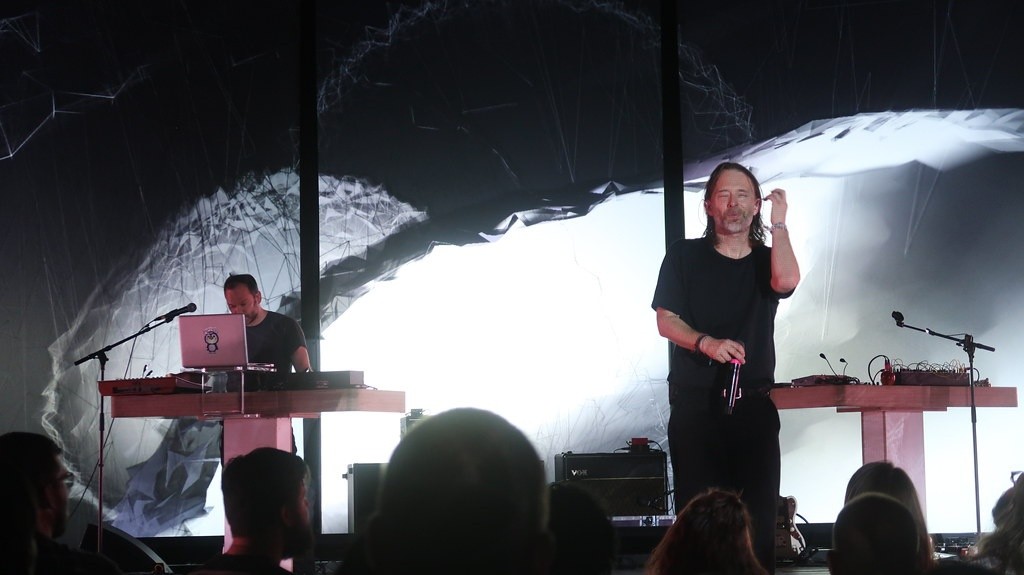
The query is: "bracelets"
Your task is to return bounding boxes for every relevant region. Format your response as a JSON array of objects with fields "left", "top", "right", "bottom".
[
  {"left": 770, "top": 222, "right": 788, "bottom": 234},
  {"left": 694, "top": 334, "right": 710, "bottom": 354}
]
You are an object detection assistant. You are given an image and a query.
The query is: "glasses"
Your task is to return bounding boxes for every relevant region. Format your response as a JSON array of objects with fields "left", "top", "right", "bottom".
[{"left": 47, "top": 472, "right": 75, "bottom": 489}]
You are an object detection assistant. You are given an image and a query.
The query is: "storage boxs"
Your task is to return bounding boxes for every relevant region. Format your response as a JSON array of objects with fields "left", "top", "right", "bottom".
[
  {"left": 347, "top": 462, "right": 387, "bottom": 535},
  {"left": 612, "top": 515, "right": 677, "bottom": 527}
]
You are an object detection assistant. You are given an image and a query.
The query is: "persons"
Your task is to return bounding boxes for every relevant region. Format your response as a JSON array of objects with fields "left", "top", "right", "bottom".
[
  {"left": 383, "top": 407, "right": 545, "bottom": 575},
  {"left": 549, "top": 481, "right": 619, "bottom": 575},
  {"left": 844, "top": 459, "right": 933, "bottom": 575},
  {"left": 651, "top": 163, "right": 800, "bottom": 575},
  {"left": 827, "top": 491, "right": 918, "bottom": 575},
  {"left": 217, "top": 271, "right": 313, "bottom": 468},
  {"left": 643, "top": 485, "right": 765, "bottom": 575},
  {"left": 191, "top": 446, "right": 316, "bottom": 575},
  {"left": 965, "top": 472, "right": 1024, "bottom": 575},
  {"left": 0, "top": 431, "right": 123, "bottom": 575}
]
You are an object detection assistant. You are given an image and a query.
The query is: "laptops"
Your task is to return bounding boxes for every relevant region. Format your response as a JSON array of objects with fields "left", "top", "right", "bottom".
[{"left": 179, "top": 313, "right": 274, "bottom": 367}]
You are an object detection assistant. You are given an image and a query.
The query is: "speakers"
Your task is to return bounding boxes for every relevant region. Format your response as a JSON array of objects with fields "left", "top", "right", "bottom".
[
  {"left": 343, "top": 463, "right": 386, "bottom": 535},
  {"left": 79, "top": 523, "right": 173, "bottom": 574},
  {"left": 554, "top": 452, "right": 669, "bottom": 516}
]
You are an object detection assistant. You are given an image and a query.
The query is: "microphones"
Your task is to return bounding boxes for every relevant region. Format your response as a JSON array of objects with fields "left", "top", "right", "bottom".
[
  {"left": 154, "top": 303, "right": 196, "bottom": 321},
  {"left": 637, "top": 497, "right": 669, "bottom": 511},
  {"left": 726, "top": 340, "right": 744, "bottom": 414}
]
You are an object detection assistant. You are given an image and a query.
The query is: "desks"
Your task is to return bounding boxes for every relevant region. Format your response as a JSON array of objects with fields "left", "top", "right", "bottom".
[
  {"left": 769, "top": 385, "right": 1017, "bottom": 545},
  {"left": 111, "top": 392, "right": 404, "bottom": 573}
]
